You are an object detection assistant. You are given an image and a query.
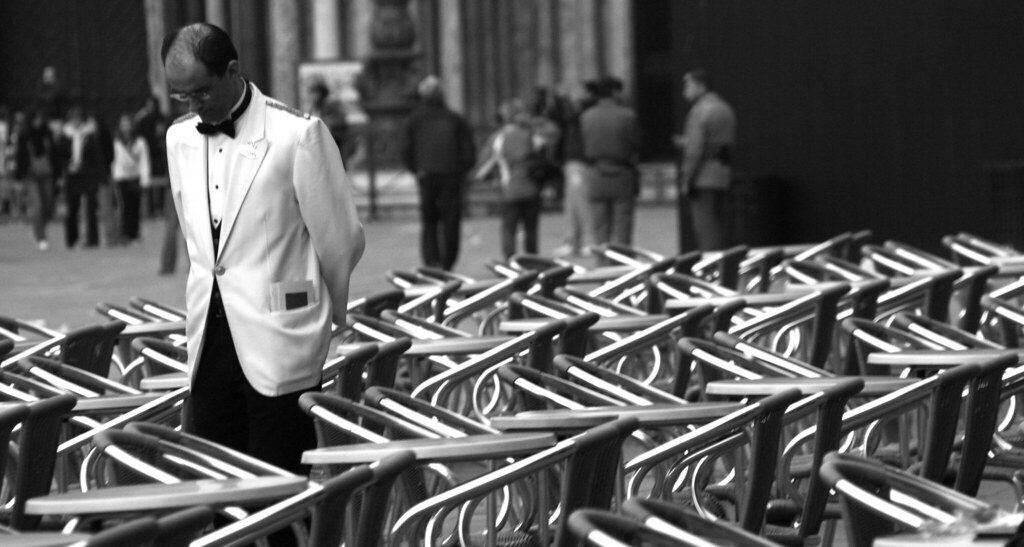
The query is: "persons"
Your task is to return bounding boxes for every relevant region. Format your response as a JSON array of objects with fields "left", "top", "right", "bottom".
[
  {"left": 470, "top": 98, "right": 562, "bottom": 261},
  {"left": 670, "top": 67, "right": 738, "bottom": 251},
  {"left": 160, "top": 24, "right": 366, "bottom": 547},
  {"left": 397, "top": 74, "right": 477, "bottom": 272},
  {"left": 306, "top": 74, "right": 357, "bottom": 172},
  {"left": 577, "top": 75, "right": 644, "bottom": 266},
  {"left": 0, "top": 97, "right": 168, "bottom": 251}
]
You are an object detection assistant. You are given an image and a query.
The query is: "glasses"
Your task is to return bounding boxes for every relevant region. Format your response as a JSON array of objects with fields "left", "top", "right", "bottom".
[{"left": 169, "top": 74, "right": 218, "bottom": 102}]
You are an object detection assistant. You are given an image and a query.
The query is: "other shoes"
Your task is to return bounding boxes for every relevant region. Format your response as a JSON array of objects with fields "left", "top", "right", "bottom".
[{"left": 37, "top": 239, "right": 49, "bottom": 251}]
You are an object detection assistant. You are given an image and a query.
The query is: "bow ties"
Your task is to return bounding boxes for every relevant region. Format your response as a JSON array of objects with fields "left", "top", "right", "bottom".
[{"left": 195, "top": 120, "right": 234, "bottom": 138}]
